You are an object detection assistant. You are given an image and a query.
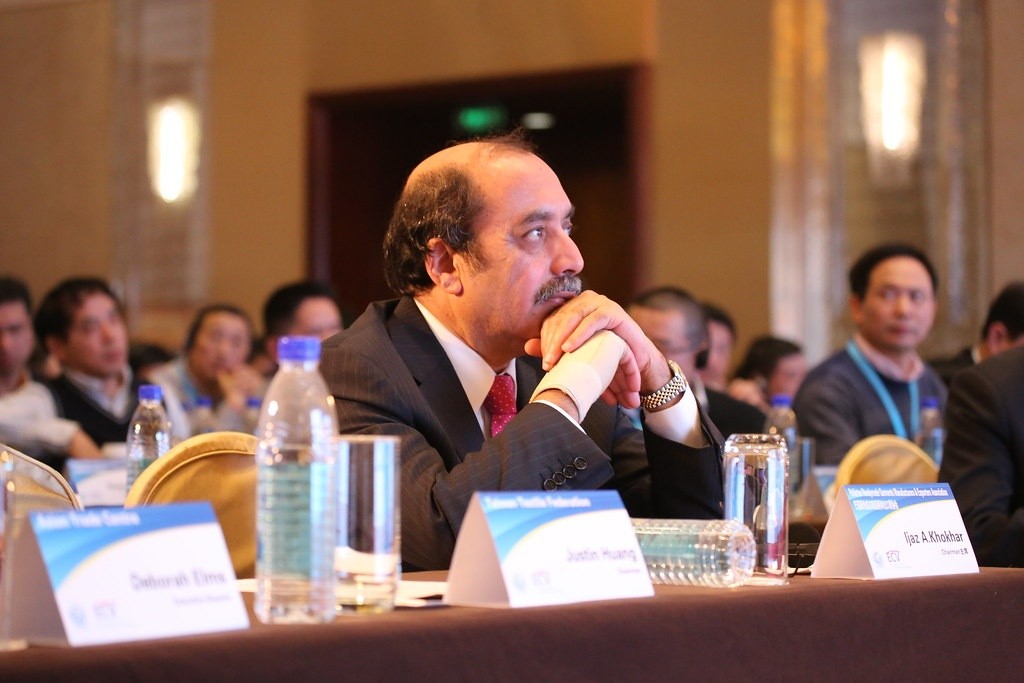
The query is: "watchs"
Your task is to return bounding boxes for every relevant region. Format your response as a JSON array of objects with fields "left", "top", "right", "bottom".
[{"left": 639, "top": 361, "right": 686, "bottom": 407}]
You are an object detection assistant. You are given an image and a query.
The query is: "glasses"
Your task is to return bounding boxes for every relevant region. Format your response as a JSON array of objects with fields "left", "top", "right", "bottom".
[{"left": 648, "top": 338, "right": 694, "bottom": 362}]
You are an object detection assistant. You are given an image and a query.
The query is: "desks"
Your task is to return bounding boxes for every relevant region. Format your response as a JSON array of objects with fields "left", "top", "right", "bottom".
[{"left": 0, "top": 565, "right": 1024, "bottom": 683}]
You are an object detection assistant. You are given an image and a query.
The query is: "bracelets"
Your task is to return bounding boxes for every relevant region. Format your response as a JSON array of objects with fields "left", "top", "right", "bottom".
[{"left": 529, "top": 363, "right": 603, "bottom": 425}]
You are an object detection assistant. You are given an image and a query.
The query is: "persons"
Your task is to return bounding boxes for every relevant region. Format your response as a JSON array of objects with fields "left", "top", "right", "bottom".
[
  {"left": 315, "top": 137, "right": 755, "bottom": 572},
  {"left": 0, "top": 242, "right": 1023, "bottom": 569}
]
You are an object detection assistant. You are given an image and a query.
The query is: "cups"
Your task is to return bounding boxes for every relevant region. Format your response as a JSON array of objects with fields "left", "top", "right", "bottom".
[
  {"left": 787, "top": 437, "right": 816, "bottom": 494},
  {"left": 336, "top": 433, "right": 403, "bottom": 609},
  {"left": 722, "top": 433, "right": 789, "bottom": 587}
]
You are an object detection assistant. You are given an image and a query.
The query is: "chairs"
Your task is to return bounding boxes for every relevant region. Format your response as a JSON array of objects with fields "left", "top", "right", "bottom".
[
  {"left": 834, "top": 434, "right": 937, "bottom": 497},
  {"left": 124, "top": 430, "right": 259, "bottom": 581}
]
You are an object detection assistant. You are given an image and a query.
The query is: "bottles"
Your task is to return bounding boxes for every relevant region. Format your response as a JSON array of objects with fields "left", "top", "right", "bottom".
[
  {"left": 629, "top": 516, "right": 756, "bottom": 589},
  {"left": 193, "top": 396, "right": 212, "bottom": 434},
  {"left": 254, "top": 335, "right": 338, "bottom": 626},
  {"left": 762, "top": 396, "right": 798, "bottom": 455},
  {"left": 124, "top": 385, "right": 169, "bottom": 501},
  {"left": 245, "top": 398, "right": 262, "bottom": 431},
  {"left": 916, "top": 396, "right": 945, "bottom": 467}
]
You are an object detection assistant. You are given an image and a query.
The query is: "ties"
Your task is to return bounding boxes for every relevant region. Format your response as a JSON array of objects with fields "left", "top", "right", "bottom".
[{"left": 483, "top": 375, "right": 520, "bottom": 438}]
